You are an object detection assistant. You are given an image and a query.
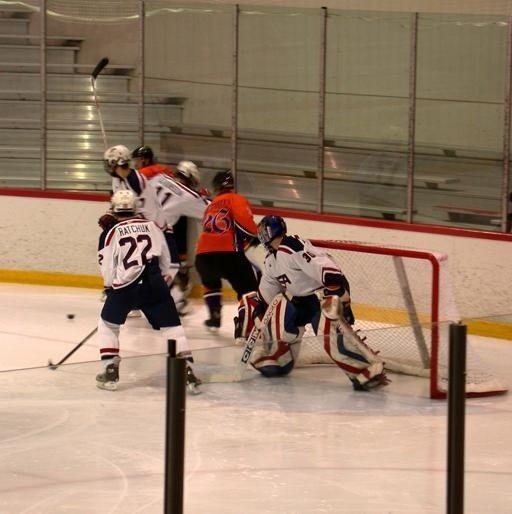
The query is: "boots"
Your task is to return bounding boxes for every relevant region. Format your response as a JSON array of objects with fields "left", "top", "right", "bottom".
[
  {"left": 234, "top": 316, "right": 243, "bottom": 339},
  {"left": 206, "top": 305, "right": 221, "bottom": 328},
  {"left": 96, "top": 356, "right": 120, "bottom": 382},
  {"left": 186, "top": 366, "right": 201, "bottom": 387}
]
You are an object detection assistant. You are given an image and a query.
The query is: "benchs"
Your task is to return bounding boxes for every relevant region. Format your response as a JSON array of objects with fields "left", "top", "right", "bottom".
[
  {"left": 1, "top": 7, "right": 186, "bottom": 194},
  {"left": 150, "top": 121, "right": 512, "bottom": 233}
]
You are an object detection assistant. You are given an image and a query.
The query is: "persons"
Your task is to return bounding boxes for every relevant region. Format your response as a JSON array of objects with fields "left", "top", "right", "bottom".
[
  {"left": 246, "top": 215, "right": 387, "bottom": 392},
  {"left": 130, "top": 146, "right": 174, "bottom": 179},
  {"left": 104, "top": 145, "right": 188, "bottom": 313},
  {"left": 94, "top": 189, "right": 203, "bottom": 389},
  {"left": 193, "top": 170, "right": 259, "bottom": 342},
  {"left": 149, "top": 161, "right": 198, "bottom": 289}
]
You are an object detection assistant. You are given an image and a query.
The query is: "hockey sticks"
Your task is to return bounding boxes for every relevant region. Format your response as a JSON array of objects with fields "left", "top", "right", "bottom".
[
  {"left": 48, "top": 326, "right": 98, "bottom": 370},
  {"left": 92, "top": 58, "right": 111, "bottom": 148}
]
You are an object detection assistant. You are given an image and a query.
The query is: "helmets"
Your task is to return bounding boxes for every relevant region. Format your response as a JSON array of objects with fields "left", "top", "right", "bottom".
[
  {"left": 111, "top": 189, "right": 137, "bottom": 214},
  {"left": 213, "top": 171, "right": 235, "bottom": 192},
  {"left": 178, "top": 161, "right": 201, "bottom": 185},
  {"left": 132, "top": 146, "right": 153, "bottom": 160},
  {"left": 256, "top": 215, "right": 286, "bottom": 250},
  {"left": 102, "top": 145, "right": 131, "bottom": 167}
]
